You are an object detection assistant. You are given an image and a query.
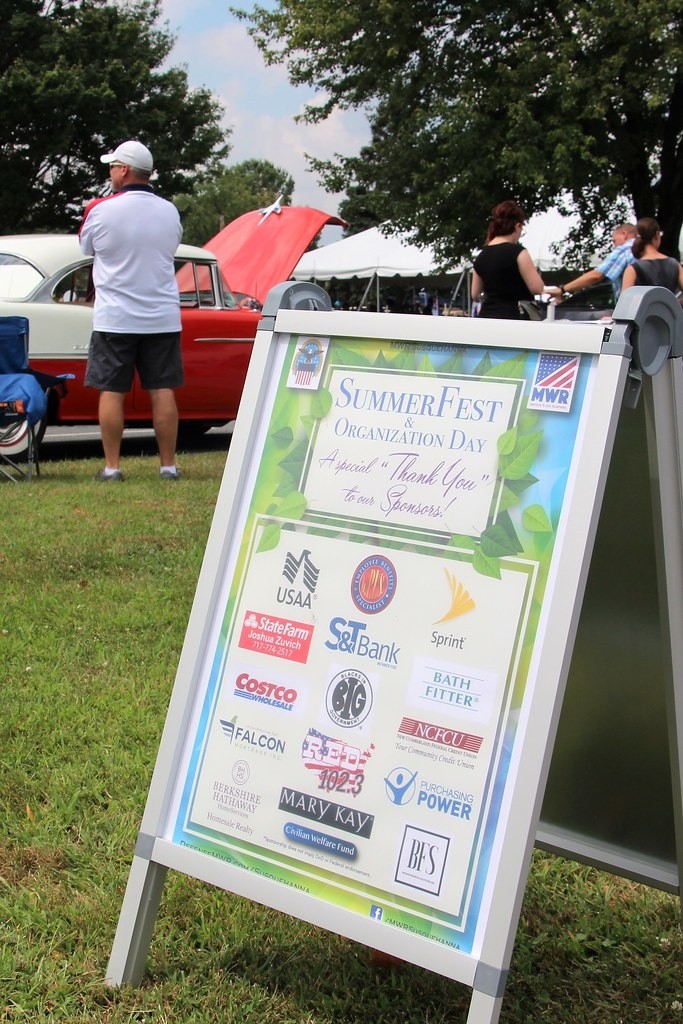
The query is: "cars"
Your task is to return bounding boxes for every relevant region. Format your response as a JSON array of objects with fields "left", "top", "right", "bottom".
[
  {"left": 518, "top": 283, "right": 615, "bottom": 322},
  {"left": 0, "top": 205, "right": 351, "bottom": 439}
]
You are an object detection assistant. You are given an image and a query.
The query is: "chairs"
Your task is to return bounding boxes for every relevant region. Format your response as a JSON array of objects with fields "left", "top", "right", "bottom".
[{"left": 0, "top": 316, "right": 76, "bottom": 483}]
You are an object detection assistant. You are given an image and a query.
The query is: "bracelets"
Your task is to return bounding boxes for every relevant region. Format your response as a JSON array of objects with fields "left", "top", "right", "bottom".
[{"left": 560, "top": 285, "right": 565, "bottom": 294}]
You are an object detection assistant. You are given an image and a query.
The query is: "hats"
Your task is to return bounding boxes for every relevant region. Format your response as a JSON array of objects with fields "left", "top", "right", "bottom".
[{"left": 100, "top": 140, "right": 152, "bottom": 171}]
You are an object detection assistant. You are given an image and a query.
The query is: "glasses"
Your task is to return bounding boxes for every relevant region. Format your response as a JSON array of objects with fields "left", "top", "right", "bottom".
[{"left": 109, "top": 162, "right": 123, "bottom": 169}]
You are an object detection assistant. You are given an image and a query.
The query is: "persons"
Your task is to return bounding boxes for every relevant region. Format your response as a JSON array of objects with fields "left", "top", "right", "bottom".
[
  {"left": 422, "top": 297, "right": 434, "bottom": 315},
  {"left": 471, "top": 202, "right": 543, "bottom": 320},
  {"left": 371, "top": 288, "right": 386, "bottom": 309},
  {"left": 544, "top": 223, "right": 637, "bottom": 305},
  {"left": 324, "top": 280, "right": 337, "bottom": 307},
  {"left": 77, "top": 141, "right": 183, "bottom": 483},
  {"left": 348, "top": 283, "right": 362, "bottom": 305},
  {"left": 622, "top": 217, "right": 683, "bottom": 294}
]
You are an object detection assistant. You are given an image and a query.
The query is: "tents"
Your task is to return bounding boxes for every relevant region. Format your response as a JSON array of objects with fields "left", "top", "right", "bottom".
[{"left": 288, "top": 134, "right": 683, "bottom": 313}]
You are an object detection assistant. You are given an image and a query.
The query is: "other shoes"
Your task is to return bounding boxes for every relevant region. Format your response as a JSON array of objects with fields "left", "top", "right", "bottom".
[
  {"left": 160, "top": 470, "right": 181, "bottom": 482},
  {"left": 91, "top": 467, "right": 122, "bottom": 482}
]
